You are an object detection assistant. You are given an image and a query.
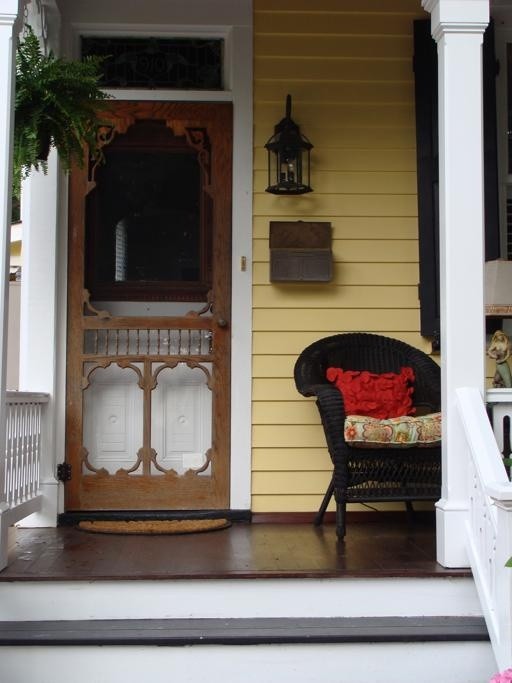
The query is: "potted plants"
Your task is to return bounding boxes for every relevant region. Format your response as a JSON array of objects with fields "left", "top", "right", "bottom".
[{"left": 12, "top": 22, "right": 118, "bottom": 200}]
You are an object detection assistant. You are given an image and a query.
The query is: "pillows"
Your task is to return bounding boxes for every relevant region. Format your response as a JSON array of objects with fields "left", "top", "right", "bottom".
[{"left": 326, "top": 364, "right": 414, "bottom": 419}]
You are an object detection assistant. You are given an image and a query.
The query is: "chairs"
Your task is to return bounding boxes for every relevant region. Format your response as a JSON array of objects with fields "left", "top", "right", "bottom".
[{"left": 294, "top": 333, "right": 441, "bottom": 543}]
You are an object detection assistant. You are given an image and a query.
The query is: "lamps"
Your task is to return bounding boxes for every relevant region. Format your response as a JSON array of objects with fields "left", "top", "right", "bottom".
[
  {"left": 264, "top": 94, "right": 314, "bottom": 195},
  {"left": 485, "top": 260, "right": 512, "bottom": 315}
]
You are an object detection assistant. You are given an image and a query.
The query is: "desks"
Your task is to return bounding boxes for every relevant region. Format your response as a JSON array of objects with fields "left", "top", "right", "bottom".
[{"left": 486, "top": 388, "right": 512, "bottom": 459}]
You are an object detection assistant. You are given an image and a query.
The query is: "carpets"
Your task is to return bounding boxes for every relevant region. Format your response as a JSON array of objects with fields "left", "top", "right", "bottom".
[{"left": 76, "top": 518, "right": 232, "bottom": 535}]
